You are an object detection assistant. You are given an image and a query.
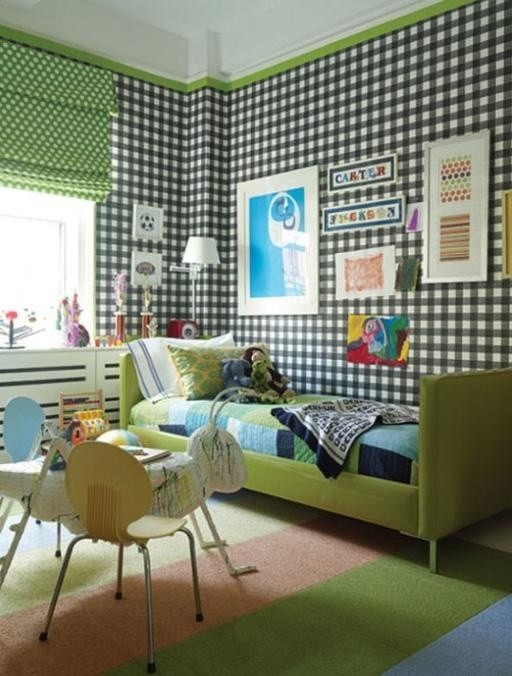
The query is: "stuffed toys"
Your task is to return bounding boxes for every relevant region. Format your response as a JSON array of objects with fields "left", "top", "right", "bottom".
[{"left": 221, "top": 346, "right": 297, "bottom": 405}]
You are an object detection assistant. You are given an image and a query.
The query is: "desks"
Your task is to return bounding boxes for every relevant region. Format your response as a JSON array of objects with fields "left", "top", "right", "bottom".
[{"left": 0, "top": 384, "right": 259, "bottom": 588}]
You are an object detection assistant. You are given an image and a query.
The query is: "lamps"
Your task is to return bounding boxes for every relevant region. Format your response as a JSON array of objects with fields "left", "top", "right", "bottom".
[{"left": 182, "top": 236, "right": 221, "bottom": 321}]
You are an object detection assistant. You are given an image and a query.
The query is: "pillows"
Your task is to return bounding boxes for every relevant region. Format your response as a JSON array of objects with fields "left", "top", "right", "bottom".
[{"left": 127, "top": 329, "right": 274, "bottom": 401}]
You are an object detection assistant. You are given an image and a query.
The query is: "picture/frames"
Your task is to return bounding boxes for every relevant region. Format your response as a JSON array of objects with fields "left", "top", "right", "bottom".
[
  {"left": 501, "top": 189, "right": 512, "bottom": 281},
  {"left": 237, "top": 166, "right": 319, "bottom": 315},
  {"left": 424, "top": 129, "right": 490, "bottom": 284},
  {"left": 334, "top": 244, "right": 396, "bottom": 298},
  {"left": 130, "top": 203, "right": 164, "bottom": 287}
]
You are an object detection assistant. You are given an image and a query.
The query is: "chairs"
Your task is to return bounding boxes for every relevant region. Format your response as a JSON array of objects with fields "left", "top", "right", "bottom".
[
  {"left": 3, "top": 397, "right": 62, "bottom": 559},
  {"left": 38, "top": 439, "right": 205, "bottom": 674}
]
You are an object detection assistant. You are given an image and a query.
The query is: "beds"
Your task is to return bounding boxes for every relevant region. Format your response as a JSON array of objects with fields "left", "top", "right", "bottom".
[{"left": 119, "top": 349, "right": 511, "bottom": 573}]
